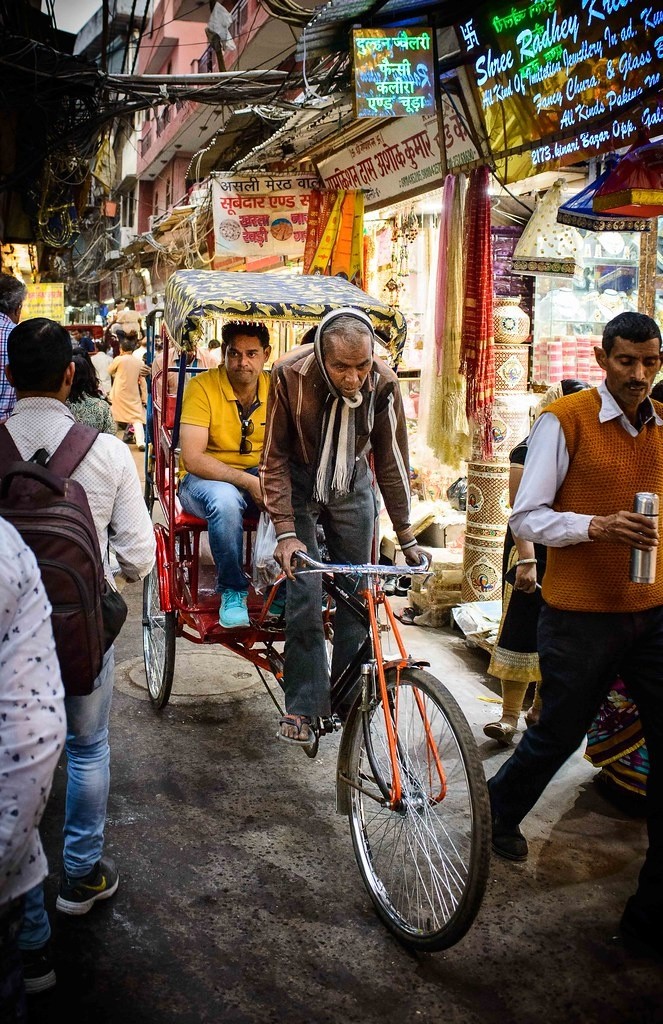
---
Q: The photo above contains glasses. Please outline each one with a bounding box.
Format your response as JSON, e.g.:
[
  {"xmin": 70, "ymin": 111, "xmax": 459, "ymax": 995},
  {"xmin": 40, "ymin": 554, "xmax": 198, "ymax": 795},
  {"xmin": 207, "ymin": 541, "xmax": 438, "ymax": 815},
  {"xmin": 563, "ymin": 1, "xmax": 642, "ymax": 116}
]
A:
[{"xmin": 239, "ymin": 419, "xmax": 254, "ymax": 454}]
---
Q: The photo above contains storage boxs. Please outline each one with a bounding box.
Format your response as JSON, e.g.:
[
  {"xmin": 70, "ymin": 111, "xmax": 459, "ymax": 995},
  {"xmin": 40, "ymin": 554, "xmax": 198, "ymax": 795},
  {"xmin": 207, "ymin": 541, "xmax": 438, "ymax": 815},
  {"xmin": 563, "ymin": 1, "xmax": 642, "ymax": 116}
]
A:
[{"xmin": 381, "ymin": 513, "xmax": 464, "ymax": 626}]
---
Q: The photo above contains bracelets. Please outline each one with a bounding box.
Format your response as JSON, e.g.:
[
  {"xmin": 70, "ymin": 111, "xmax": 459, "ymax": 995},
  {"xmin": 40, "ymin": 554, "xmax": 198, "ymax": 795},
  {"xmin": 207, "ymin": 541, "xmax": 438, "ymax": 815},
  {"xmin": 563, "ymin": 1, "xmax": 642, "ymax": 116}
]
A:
[{"xmin": 515, "ymin": 558, "xmax": 537, "ymax": 566}]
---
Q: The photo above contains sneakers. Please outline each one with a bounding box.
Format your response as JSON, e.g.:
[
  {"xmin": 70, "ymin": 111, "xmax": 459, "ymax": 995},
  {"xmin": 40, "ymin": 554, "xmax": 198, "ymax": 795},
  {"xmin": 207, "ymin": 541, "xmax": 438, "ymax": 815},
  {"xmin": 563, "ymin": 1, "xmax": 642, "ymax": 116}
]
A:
[
  {"xmin": 219, "ymin": 589, "xmax": 251, "ymax": 628},
  {"xmin": 23, "ymin": 945, "xmax": 56, "ymax": 993},
  {"xmin": 57, "ymin": 859, "xmax": 119, "ymax": 915},
  {"xmin": 263, "ymin": 584, "xmax": 337, "ymax": 616}
]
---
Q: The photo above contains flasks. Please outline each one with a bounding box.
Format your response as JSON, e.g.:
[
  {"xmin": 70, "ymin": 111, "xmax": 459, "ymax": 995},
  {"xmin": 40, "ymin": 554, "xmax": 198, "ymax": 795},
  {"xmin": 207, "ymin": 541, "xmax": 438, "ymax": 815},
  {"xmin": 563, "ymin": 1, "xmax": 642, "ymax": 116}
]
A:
[{"xmin": 631, "ymin": 491, "xmax": 658, "ymax": 584}]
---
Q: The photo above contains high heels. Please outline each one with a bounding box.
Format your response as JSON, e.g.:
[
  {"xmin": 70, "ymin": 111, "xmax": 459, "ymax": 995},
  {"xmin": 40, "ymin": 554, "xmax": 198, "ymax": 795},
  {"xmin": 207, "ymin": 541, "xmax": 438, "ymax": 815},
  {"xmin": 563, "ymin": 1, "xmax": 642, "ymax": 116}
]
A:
[
  {"xmin": 483, "ymin": 721, "xmax": 517, "ymax": 745},
  {"xmin": 524, "ymin": 715, "xmax": 539, "ymax": 728}
]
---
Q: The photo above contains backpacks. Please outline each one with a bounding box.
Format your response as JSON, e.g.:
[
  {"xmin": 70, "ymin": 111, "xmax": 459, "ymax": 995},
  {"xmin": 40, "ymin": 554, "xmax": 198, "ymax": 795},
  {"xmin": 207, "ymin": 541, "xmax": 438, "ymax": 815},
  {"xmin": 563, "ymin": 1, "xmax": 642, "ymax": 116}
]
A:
[{"xmin": 0, "ymin": 424, "xmax": 127, "ymax": 696}]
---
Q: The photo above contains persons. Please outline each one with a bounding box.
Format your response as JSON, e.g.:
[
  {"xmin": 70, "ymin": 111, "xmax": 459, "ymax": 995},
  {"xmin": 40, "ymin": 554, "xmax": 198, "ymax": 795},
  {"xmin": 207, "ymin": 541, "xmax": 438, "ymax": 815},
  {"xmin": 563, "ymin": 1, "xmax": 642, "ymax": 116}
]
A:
[
  {"xmin": 175, "ymin": 322, "xmax": 272, "ymax": 628},
  {"xmin": 0, "ymin": 270, "xmax": 221, "ymax": 1024},
  {"xmin": 482, "ymin": 312, "xmax": 663, "ymax": 964},
  {"xmin": 258, "ymin": 310, "xmax": 432, "ymax": 746}
]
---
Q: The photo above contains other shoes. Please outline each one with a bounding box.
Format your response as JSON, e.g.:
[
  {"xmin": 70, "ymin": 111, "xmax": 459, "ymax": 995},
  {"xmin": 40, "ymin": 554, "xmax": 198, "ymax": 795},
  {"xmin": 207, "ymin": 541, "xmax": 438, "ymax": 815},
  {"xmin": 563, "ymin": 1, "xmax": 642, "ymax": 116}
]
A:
[
  {"xmin": 396, "ymin": 575, "xmax": 413, "ymax": 595},
  {"xmin": 382, "ymin": 574, "xmax": 401, "ymax": 595},
  {"xmin": 618, "ymin": 896, "xmax": 662, "ymax": 960},
  {"xmin": 492, "ymin": 813, "xmax": 529, "ymax": 861},
  {"xmin": 123, "ymin": 426, "xmax": 131, "ymax": 440},
  {"xmin": 136, "ymin": 442, "xmax": 146, "ymax": 452}
]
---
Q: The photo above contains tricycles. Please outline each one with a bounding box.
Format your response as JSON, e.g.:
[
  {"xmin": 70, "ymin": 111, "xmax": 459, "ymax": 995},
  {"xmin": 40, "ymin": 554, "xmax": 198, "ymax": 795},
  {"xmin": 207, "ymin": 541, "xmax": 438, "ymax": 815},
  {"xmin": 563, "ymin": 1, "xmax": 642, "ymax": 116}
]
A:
[{"xmin": 135, "ymin": 268, "xmax": 492, "ymax": 950}]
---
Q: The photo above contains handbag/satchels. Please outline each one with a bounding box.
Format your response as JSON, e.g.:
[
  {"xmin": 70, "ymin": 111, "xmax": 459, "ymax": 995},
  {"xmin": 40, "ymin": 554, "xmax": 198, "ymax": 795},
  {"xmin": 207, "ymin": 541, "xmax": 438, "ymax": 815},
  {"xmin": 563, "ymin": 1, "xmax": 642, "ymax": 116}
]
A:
[{"xmin": 252, "ymin": 511, "xmax": 282, "ymax": 591}]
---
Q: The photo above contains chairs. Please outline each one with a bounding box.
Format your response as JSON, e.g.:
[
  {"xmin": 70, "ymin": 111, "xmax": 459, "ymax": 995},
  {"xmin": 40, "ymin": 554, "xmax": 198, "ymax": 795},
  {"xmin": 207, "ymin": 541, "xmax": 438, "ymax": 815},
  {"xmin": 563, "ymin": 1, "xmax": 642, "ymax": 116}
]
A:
[{"xmin": 152, "ymin": 367, "xmax": 260, "ymax": 605}]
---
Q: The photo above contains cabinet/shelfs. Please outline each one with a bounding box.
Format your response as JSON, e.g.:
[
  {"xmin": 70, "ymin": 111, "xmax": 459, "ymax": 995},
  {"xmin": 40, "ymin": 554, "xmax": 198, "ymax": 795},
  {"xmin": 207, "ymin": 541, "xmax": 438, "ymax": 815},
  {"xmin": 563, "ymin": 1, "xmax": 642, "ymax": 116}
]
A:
[{"xmin": 533, "ymin": 231, "xmax": 663, "ymax": 389}]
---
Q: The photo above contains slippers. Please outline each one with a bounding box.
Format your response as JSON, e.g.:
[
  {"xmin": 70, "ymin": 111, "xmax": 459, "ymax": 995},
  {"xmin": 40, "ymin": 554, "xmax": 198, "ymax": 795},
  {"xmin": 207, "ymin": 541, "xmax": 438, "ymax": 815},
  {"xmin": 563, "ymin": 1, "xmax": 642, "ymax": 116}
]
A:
[
  {"xmin": 277, "ymin": 715, "xmax": 313, "ymax": 746},
  {"xmin": 394, "ymin": 607, "xmax": 416, "ymax": 624}
]
